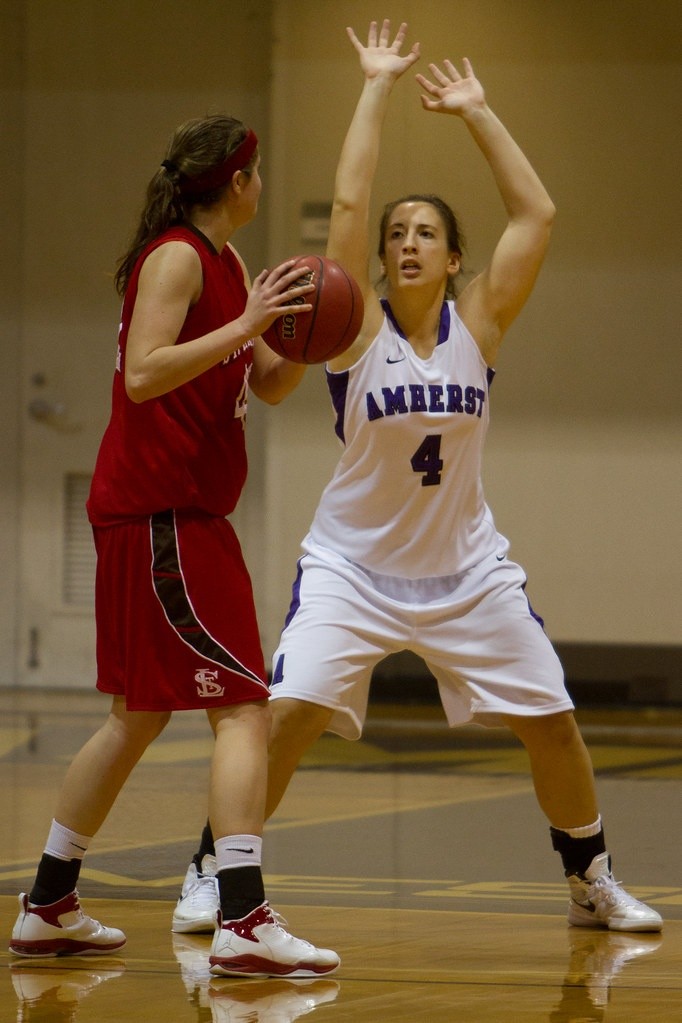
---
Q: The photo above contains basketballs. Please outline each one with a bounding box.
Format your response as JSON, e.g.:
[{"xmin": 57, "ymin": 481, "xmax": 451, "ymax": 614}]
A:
[{"xmin": 259, "ymin": 256, "xmax": 364, "ymax": 365}]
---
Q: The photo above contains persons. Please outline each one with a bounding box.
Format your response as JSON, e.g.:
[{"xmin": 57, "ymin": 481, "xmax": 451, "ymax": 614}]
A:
[
  {"xmin": 2, "ymin": 113, "xmax": 346, "ymax": 977},
  {"xmin": 172, "ymin": 16, "xmax": 667, "ymax": 941}
]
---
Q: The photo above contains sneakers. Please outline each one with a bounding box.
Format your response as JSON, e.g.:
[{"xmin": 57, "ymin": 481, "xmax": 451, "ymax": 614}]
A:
[
  {"xmin": 169, "ymin": 853, "xmax": 219, "ymax": 931},
  {"xmin": 169, "ymin": 933, "xmax": 213, "ymax": 974},
  {"xmin": 8, "ymin": 957, "xmax": 128, "ymax": 1022},
  {"xmin": 7, "ymin": 886, "xmax": 128, "ymax": 956},
  {"xmin": 567, "ymin": 927, "xmax": 664, "ymax": 977},
  {"xmin": 566, "ymin": 852, "xmax": 664, "ymax": 930},
  {"xmin": 209, "ymin": 899, "xmax": 341, "ymax": 978},
  {"xmin": 208, "ymin": 978, "xmax": 340, "ymax": 1023}
]
